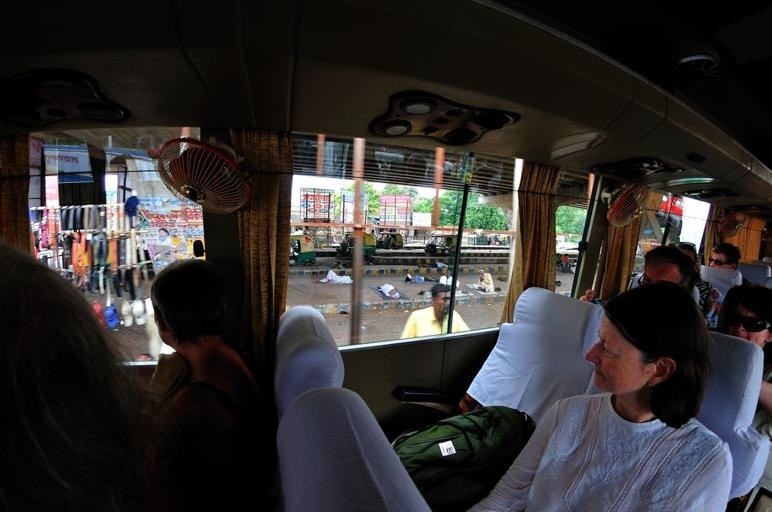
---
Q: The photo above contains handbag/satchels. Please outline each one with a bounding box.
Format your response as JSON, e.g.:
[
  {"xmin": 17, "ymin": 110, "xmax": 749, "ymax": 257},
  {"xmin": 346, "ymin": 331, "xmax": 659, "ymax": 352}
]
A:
[{"xmin": 391, "ymin": 406, "xmax": 536, "ymax": 512}]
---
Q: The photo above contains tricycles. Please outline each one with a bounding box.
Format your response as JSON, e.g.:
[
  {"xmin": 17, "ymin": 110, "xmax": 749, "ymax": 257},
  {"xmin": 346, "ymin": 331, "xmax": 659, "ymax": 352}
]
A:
[
  {"xmin": 333, "ymin": 231, "xmax": 378, "ymax": 259},
  {"xmin": 377, "ymin": 231, "xmax": 404, "ymax": 250},
  {"xmin": 467, "ymin": 232, "xmax": 487, "ymax": 245},
  {"xmin": 422, "ymin": 232, "xmax": 457, "ymax": 255},
  {"xmin": 288, "ymin": 232, "xmax": 316, "ymax": 267}
]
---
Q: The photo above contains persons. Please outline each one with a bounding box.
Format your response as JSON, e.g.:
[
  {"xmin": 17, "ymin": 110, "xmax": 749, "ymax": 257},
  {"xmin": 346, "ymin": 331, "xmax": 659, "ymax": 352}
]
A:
[
  {"xmin": 145, "ymin": 257, "xmax": 264, "ymax": 512},
  {"xmin": 641, "ymin": 244, "xmax": 700, "ymax": 296},
  {"xmin": 157, "ymin": 226, "xmax": 170, "ymax": 242},
  {"xmin": 715, "ymin": 281, "xmax": 772, "ymax": 419},
  {"xmin": 561, "ymin": 254, "xmax": 576, "ymax": 267},
  {"xmin": 707, "ymin": 242, "xmax": 743, "ymax": 274},
  {"xmin": 399, "ymin": 284, "xmax": 472, "ymax": 339},
  {"xmin": 0, "ymin": 249, "xmax": 171, "ymax": 511},
  {"xmin": 464, "ymin": 279, "xmax": 734, "ymax": 512},
  {"xmin": 291, "ymin": 214, "xmax": 501, "ymax": 304}
]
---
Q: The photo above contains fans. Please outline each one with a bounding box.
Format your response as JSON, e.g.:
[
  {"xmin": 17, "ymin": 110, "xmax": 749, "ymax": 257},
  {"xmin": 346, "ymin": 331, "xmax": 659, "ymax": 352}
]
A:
[
  {"xmin": 599, "ymin": 175, "xmax": 650, "ymax": 229},
  {"xmin": 157, "ymin": 139, "xmax": 253, "ymax": 213},
  {"xmin": 713, "ymin": 204, "xmax": 747, "ymax": 238}
]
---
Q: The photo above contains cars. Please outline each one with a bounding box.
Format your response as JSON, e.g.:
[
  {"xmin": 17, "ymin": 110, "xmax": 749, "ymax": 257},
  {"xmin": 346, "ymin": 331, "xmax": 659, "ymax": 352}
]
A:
[{"xmin": 556, "ymin": 244, "xmax": 580, "ymax": 254}]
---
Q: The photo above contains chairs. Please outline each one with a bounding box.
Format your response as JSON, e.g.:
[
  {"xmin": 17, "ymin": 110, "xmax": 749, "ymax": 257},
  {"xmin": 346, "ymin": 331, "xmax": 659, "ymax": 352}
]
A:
[
  {"xmin": 274, "ymin": 306, "xmax": 347, "ymax": 415},
  {"xmin": 374, "ymin": 286, "xmax": 609, "ymax": 504},
  {"xmin": 277, "ymin": 392, "xmax": 432, "ymax": 512},
  {"xmin": 587, "ymin": 256, "xmax": 771, "ymax": 511}
]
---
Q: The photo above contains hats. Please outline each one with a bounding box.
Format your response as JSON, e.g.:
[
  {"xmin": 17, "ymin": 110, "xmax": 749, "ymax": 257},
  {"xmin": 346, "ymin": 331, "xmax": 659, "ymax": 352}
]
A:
[{"xmin": 93, "ymin": 298, "xmax": 154, "ymax": 328}]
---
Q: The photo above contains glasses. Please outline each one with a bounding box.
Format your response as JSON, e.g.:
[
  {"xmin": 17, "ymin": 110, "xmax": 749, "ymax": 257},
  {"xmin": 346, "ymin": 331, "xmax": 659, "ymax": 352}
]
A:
[
  {"xmin": 708, "ymin": 255, "xmax": 729, "ymax": 265},
  {"xmin": 722, "ymin": 309, "xmax": 772, "ymax": 332}
]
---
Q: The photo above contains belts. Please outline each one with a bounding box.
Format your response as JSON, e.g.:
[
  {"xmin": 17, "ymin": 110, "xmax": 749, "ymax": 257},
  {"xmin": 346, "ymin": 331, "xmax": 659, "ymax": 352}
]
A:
[{"xmin": 34, "ymin": 204, "xmax": 138, "ymax": 306}]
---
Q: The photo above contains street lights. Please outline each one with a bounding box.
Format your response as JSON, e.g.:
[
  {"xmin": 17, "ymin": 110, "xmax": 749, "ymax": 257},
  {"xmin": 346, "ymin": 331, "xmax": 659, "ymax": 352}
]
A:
[{"xmin": 108, "ymin": 154, "xmax": 129, "ymax": 202}]
